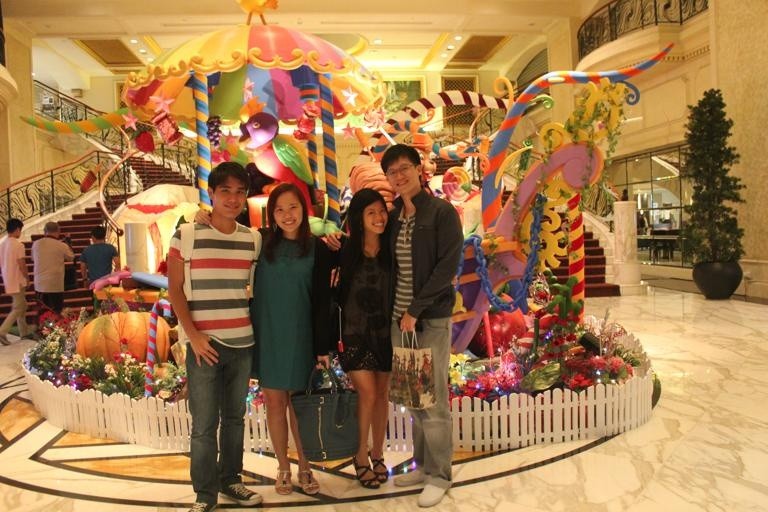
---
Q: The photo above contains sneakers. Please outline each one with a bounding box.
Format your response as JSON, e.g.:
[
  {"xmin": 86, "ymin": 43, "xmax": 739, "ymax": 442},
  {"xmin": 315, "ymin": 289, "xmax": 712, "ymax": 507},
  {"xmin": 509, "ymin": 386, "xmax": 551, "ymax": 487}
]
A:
[
  {"xmin": 393, "ymin": 469, "xmax": 427, "ymax": 487},
  {"xmin": 218, "ymin": 483, "xmax": 263, "ymax": 506},
  {"xmin": 189, "ymin": 502, "xmax": 217, "ymax": 512},
  {"xmin": 417, "ymin": 483, "xmax": 452, "ymax": 507}
]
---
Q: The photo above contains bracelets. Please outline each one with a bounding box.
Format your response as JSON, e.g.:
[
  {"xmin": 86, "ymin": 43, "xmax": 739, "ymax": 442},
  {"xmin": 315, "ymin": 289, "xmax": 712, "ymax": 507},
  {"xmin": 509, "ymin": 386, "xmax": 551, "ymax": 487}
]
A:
[{"xmin": 83, "ymin": 277, "xmax": 88, "ymax": 281}]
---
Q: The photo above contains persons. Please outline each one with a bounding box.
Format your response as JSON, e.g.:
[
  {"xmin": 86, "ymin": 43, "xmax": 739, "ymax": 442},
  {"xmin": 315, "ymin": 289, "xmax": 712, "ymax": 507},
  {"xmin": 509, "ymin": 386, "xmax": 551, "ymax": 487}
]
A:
[
  {"xmin": 327, "ymin": 143, "xmax": 467, "ymax": 507},
  {"xmin": 0, "ymin": 218, "xmax": 36, "ymax": 345},
  {"xmin": 323, "ymin": 187, "xmax": 460, "ymax": 490},
  {"xmin": 193, "ymin": 182, "xmax": 334, "ymax": 497},
  {"xmin": 31, "ymin": 222, "xmax": 75, "ymax": 327},
  {"xmin": 166, "ymin": 160, "xmax": 266, "ymax": 510},
  {"xmin": 78, "ymin": 226, "xmax": 121, "ymax": 310}
]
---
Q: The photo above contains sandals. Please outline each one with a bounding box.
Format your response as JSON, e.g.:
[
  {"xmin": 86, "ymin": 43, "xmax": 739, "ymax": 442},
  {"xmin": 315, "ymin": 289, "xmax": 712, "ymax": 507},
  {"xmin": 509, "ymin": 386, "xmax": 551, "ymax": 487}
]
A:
[
  {"xmin": 275, "ymin": 470, "xmax": 293, "ymax": 495},
  {"xmin": 353, "ymin": 456, "xmax": 380, "ymax": 489},
  {"xmin": 371, "ymin": 458, "xmax": 389, "ymax": 483},
  {"xmin": 299, "ymin": 470, "xmax": 319, "ymax": 495}
]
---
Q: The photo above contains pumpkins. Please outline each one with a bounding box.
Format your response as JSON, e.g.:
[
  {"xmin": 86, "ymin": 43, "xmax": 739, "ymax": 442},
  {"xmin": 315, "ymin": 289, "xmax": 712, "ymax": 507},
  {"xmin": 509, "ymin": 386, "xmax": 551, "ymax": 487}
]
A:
[{"xmin": 76, "ymin": 311, "xmax": 172, "ymax": 363}]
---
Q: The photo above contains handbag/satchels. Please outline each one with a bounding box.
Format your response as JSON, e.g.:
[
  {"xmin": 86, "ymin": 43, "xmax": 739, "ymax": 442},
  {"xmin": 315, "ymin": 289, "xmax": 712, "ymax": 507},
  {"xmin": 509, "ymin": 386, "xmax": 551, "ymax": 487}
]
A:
[
  {"xmin": 388, "ymin": 328, "xmax": 437, "ymax": 410},
  {"xmin": 291, "ymin": 361, "xmax": 361, "ymax": 462}
]
